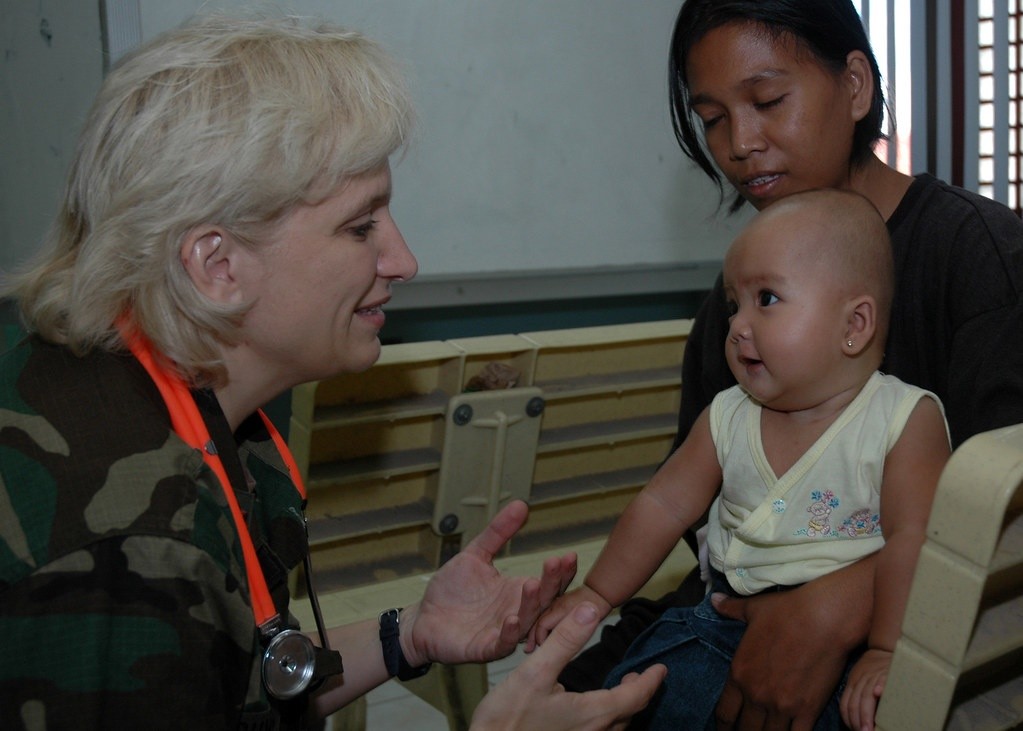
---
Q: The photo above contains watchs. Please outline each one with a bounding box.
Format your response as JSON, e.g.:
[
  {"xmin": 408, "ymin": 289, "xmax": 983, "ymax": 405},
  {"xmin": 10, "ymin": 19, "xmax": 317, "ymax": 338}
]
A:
[{"xmin": 378, "ymin": 606, "xmax": 431, "ymax": 684}]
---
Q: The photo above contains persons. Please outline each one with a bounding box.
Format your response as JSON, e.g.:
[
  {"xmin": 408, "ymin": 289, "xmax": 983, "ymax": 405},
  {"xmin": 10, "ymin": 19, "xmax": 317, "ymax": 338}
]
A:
[
  {"xmin": 518, "ymin": 187, "xmax": 953, "ymax": 731},
  {"xmin": 3, "ymin": 18, "xmax": 579, "ymax": 730},
  {"xmin": 551, "ymin": 0, "xmax": 1022, "ymax": 731}
]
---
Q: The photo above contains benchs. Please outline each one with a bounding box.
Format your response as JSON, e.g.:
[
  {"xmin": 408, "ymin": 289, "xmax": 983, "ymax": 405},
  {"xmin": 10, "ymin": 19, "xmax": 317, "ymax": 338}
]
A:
[{"xmin": 880, "ymin": 420, "xmax": 1023, "ymax": 731}]
